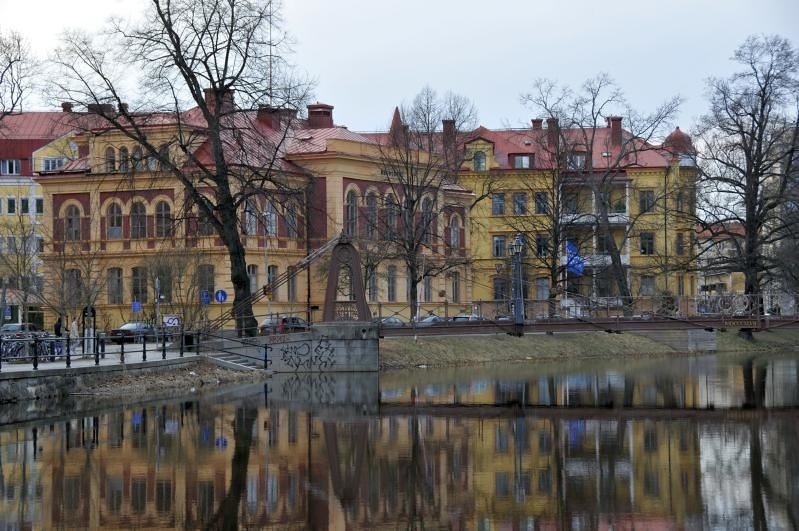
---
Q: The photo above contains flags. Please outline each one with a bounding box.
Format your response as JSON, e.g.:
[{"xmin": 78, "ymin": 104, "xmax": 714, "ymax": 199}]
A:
[{"xmin": 566, "ymin": 241, "xmax": 584, "ymax": 276}]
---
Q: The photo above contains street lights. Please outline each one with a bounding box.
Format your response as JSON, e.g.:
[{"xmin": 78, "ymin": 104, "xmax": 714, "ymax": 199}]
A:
[{"xmin": 507, "ymin": 236, "xmax": 524, "ymax": 320}]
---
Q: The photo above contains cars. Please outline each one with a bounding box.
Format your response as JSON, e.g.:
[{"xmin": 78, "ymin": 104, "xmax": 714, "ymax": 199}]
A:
[
  {"xmin": 373, "ymin": 317, "xmax": 404, "ymax": 323},
  {"xmin": 257, "ymin": 316, "xmax": 308, "ymax": 336},
  {"xmin": 110, "ymin": 323, "xmax": 152, "ymax": 345},
  {"xmin": 1, "ymin": 323, "xmax": 48, "ymax": 337}
]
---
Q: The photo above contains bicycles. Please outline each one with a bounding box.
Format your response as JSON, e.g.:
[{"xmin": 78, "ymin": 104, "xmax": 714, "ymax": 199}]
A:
[{"xmin": 0, "ymin": 332, "xmax": 63, "ymax": 362}]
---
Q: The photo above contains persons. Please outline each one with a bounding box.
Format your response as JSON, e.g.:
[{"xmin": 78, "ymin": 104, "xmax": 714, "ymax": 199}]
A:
[{"xmin": 54, "ymin": 315, "xmax": 80, "ymax": 356}]
